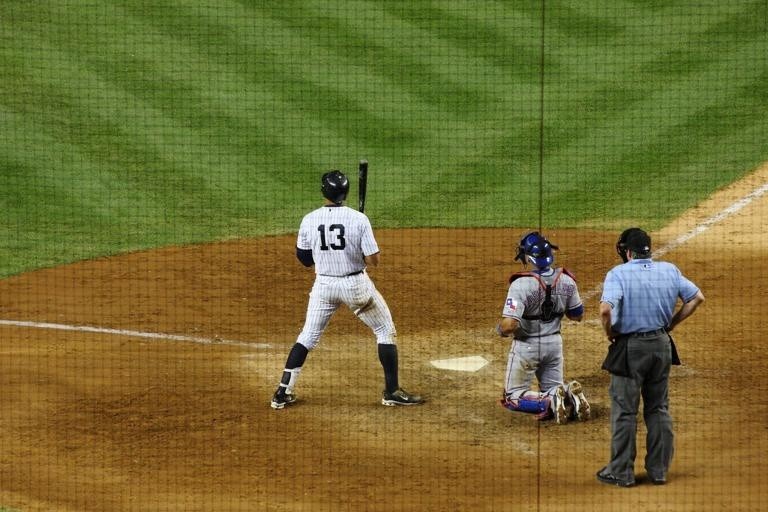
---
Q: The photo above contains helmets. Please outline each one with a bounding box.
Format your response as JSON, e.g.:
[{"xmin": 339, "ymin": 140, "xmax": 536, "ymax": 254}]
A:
[
  {"xmin": 515, "ymin": 232, "xmax": 560, "ymax": 268},
  {"xmin": 321, "ymin": 170, "xmax": 349, "ymax": 205},
  {"xmin": 616, "ymin": 227, "xmax": 650, "ymax": 262}
]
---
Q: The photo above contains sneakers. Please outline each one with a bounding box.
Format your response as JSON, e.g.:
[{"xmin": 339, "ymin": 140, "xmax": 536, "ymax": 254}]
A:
[
  {"xmin": 597, "ymin": 467, "xmax": 635, "ymax": 487},
  {"xmin": 567, "ymin": 380, "xmax": 591, "ymax": 422},
  {"xmin": 652, "ymin": 475, "xmax": 666, "ymax": 484},
  {"xmin": 549, "ymin": 386, "xmax": 569, "ymax": 425},
  {"xmin": 271, "ymin": 392, "xmax": 298, "ymax": 410},
  {"xmin": 381, "ymin": 388, "xmax": 423, "ymax": 407}
]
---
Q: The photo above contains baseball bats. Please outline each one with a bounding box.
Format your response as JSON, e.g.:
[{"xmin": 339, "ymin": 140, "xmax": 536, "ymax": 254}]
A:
[{"xmin": 357, "ymin": 160, "xmax": 369, "ymax": 213}]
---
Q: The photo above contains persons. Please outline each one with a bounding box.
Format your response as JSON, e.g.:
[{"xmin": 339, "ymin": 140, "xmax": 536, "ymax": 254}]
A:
[
  {"xmin": 496, "ymin": 231, "xmax": 591, "ymax": 426},
  {"xmin": 270, "ymin": 170, "xmax": 424, "ymax": 410},
  {"xmin": 595, "ymin": 227, "xmax": 706, "ymax": 488}
]
